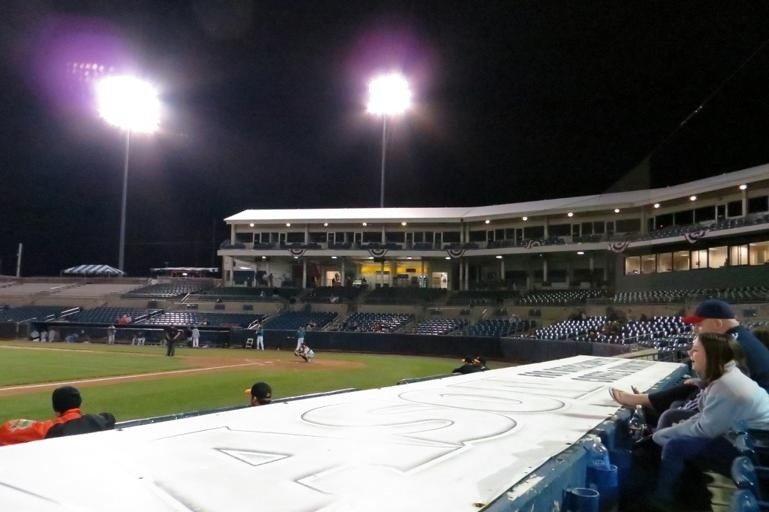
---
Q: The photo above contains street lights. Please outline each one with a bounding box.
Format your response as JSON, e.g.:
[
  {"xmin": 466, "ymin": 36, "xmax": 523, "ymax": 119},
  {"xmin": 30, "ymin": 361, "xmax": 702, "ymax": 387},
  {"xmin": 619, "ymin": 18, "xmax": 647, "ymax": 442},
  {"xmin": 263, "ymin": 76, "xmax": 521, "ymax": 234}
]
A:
[
  {"xmin": 366, "ymin": 70, "xmax": 412, "ymax": 208},
  {"xmin": 97, "ymin": 71, "xmax": 163, "ymax": 275}
]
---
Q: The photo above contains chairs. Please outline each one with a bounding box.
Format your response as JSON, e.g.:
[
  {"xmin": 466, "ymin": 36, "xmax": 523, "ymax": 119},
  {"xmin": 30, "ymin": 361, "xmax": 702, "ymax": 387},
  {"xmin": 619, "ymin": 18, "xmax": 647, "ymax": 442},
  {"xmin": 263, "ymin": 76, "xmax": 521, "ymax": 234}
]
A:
[
  {"xmin": 124, "ymin": 281, "xmax": 211, "ymax": 298},
  {"xmin": 516, "ymin": 285, "xmax": 769, "ymax": 307},
  {"xmin": 701, "ymin": 429, "xmax": 769, "ymax": 512}
]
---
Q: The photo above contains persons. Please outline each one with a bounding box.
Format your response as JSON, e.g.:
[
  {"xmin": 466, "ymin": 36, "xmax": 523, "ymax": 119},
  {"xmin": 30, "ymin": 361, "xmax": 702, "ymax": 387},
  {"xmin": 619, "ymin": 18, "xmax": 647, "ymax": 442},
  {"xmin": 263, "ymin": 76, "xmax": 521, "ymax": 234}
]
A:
[
  {"xmin": 571, "ymin": 279, "xmax": 616, "ymax": 302},
  {"xmin": 0, "ymin": 386, "xmax": 115, "ymax": 445},
  {"xmin": 294, "ymin": 326, "xmax": 314, "ymax": 362},
  {"xmin": 566, "ymin": 310, "xmax": 590, "ymax": 322},
  {"xmin": 307, "ymin": 320, "xmax": 315, "ymax": 330},
  {"xmin": 31, "ymin": 328, "xmax": 90, "ymax": 344},
  {"xmin": 454, "ymin": 356, "xmax": 489, "ymax": 374},
  {"xmin": 257, "ymin": 272, "xmax": 281, "ymax": 297},
  {"xmin": 311, "ymin": 273, "xmax": 368, "ymax": 297},
  {"xmin": 478, "ymin": 303, "xmax": 542, "ymax": 330},
  {"xmin": 164, "ymin": 323, "xmax": 181, "ymax": 357},
  {"xmin": 192, "ymin": 325, "xmax": 199, "ymax": 348},
  {"xmin": 224, "ymin": 326, "xmax": 265, "ymax": 349},
  {"xmin": 375, "ymin": 323, "xmax": 383, "ymax": 333},
  {"xmin": 474, "ymin": 278, "xmax": 552, "ymax": 292},
  {"xmin": 586, "ymin": 305, "xmax": 648, "ymax": 342},
  {"xmin": 107, "ymin": 315, "xmax": 146, "ymax": 345},
  {"xmin": 607, "ymin": 299, "xmax": 768, "ymax": 511},
  {"xmin": 244, "ymin": 382, "xmax": 272, "ymax": 406},
  {"xmin": 431, "ymin": 304, "xmax": 472, "ymax": 317},
  {"xmin": 203, "ymin": 318, "xmax": 208, "ymax": 325}
]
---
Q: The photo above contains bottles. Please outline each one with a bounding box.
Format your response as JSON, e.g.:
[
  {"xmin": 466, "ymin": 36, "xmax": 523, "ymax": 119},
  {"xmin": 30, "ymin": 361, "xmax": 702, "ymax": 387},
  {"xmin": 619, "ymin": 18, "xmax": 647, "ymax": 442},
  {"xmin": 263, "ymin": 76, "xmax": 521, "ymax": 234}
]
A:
[
  {"xmin": 625, "ymin": 414, "xmax": 640, "ymax": 445},
  {"xmin": 592, "ymin": 436, "xmax": 612, "ymax": 473},
  {"xmin": 633, "ymin": 405, "xmax": 648, "ymax": 435}
]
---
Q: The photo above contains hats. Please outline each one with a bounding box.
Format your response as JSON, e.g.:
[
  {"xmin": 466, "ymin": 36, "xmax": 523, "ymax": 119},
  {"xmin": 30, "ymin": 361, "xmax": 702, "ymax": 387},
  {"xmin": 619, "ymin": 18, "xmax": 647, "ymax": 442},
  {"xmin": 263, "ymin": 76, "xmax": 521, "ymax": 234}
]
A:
[
  {"xmin": 245, "ymin": 383, "xmax": 272, "ymax": 398},
  {"xmin": 53, "ymin": 386, "xmax": 81, "ymax": 411},
  {"xmin": 682, "ymin": 300, "xmax": 734, "ymax": 322},
  {"xmin": 462, "ymin": 355, "xmax": 481, "ymax": 363}
]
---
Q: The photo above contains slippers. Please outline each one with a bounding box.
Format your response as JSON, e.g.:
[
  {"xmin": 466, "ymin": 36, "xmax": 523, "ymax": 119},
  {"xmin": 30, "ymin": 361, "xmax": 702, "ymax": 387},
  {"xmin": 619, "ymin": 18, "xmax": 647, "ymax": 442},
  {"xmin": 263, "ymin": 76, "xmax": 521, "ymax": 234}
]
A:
[{"xmin": 609, "ymin": 385, "xmax": 640, "ymax": 409}]
